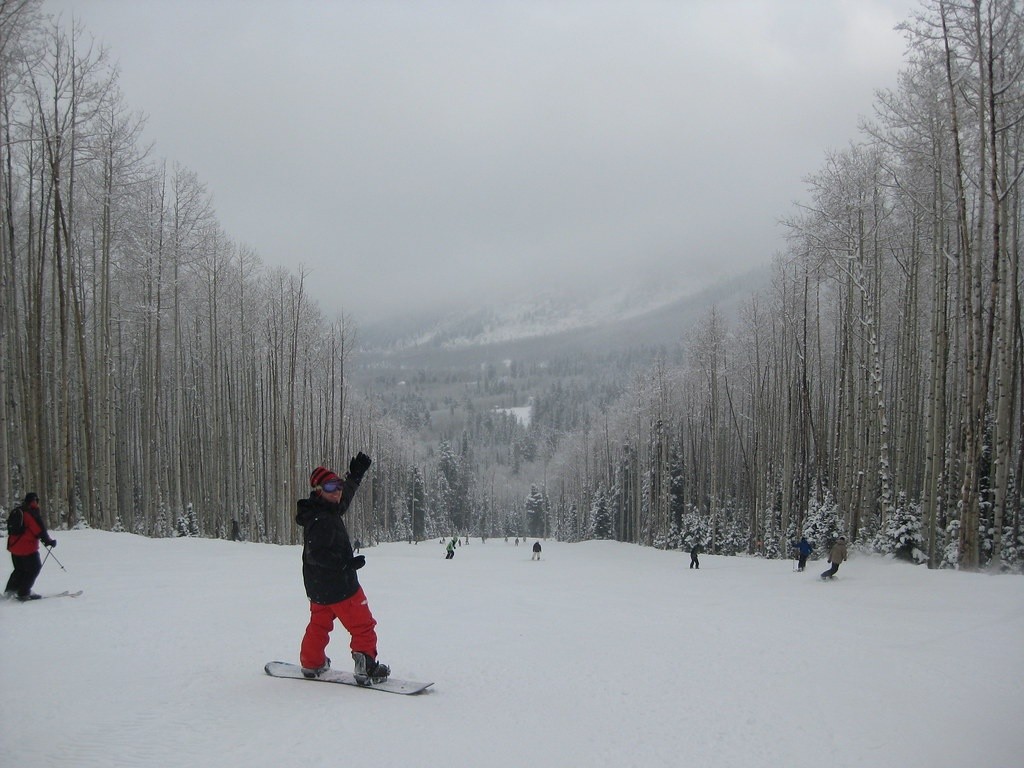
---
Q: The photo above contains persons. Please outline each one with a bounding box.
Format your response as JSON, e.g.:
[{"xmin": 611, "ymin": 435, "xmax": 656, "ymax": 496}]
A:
[
  {"xmin": 690, "ymin": 544, "xmax": 699, "ymax": 570},
  {"xmin": 531, "ymin": 541, "xmax": 541, "ymax": 560},
  {"xmin": 515, "ymin": 538, "xmax": 520, "ymax": 546},
  {"xmin": 523, "ymin": 536, "xmax": 526, "ymax": 542},
  {"xmin": 505, "ymin": 535, "xmax": 508, "ymax": 542},
  {"xmin": 230, "ymin": 518, "xmax": 242, "ymax": 542},
  {"xmin": 295, "ymin": 452, "xmax": 391, "ymax": 686},
  {"xmin": 353, "ymin": 539, "xmax": 360, "ymax": 553},
  {"xmin": 3, "ymin": 491, "xmax": 57, "ymax": 601},
  {"xmin": 820, "ymin": 537, "xmax": 848, "ymax": 581},
  {"xmin": 465, "ymin": 533, "xmax": 469, "ymax": 545},
  {"xmin": 482, "ymin": 536, "xmax": 486, "ymax": 543},
  {"xmin": 792, "ymin": 537, "xmax": 813, "ymax": 572},
  {"xmin": 445, "ymin": 536, "xmax": 458, "ymax": 559}
]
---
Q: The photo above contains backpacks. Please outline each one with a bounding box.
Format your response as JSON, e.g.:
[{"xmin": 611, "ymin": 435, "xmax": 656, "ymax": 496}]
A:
[{"xmin": 7, "ymin": 507, "xmax": 24, "ymax": 535}]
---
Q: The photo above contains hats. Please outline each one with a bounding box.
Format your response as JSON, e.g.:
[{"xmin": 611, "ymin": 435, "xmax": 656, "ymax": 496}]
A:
[{"xmin": 310, "ymin": 467, "xmax": 339, "ymax": 498}]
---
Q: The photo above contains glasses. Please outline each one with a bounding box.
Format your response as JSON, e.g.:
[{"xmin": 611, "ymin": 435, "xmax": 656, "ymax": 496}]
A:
[
  {"xmin": 322, "ymin": 479, "xmax": 345, "ymax": 493},
  {"xmin": 34, "ymin": 498, "xmax": 39, "ymax": 503}
]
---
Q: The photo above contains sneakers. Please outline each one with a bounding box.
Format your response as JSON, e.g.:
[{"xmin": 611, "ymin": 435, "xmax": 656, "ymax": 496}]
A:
[
  {"xmin": 357, "ymin": 656, "xmax": 391, "ymax": 686},
  {"xmin": 303, "ymin": 658, "xmax": 331, "ymax": 678}
]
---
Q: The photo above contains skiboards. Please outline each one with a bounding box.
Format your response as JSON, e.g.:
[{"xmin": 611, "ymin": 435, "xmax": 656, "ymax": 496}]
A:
[{"xmin": 41, "ymin": 589, "xmax": 84, "ymax": 600}]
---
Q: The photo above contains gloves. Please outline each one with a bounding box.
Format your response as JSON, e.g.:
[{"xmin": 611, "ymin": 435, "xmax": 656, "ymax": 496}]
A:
[
  {"xmin": 347, "ymin": 452, "xmax": 371, "ymax": 484},
  {"xmin": 49, "ymin": 540, "xmax": 56, "ymax": 547},
  {"xmin": 345, "ymin": 555, "xmax": 366, "ymax": 571}
]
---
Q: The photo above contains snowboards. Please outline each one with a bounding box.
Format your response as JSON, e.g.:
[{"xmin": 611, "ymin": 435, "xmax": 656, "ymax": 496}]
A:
[{"xmin": 264, "ymin": 661, "xmax": 435, "ymax": 696}]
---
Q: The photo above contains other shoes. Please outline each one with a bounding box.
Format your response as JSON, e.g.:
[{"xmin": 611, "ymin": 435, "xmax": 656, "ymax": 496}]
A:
[{"xmin": 19, "ymin": 593, "xmax": 41, "ymax": 600}]
